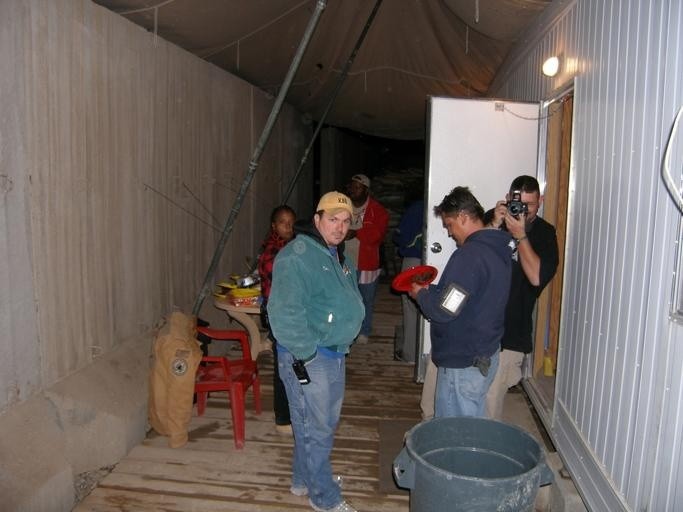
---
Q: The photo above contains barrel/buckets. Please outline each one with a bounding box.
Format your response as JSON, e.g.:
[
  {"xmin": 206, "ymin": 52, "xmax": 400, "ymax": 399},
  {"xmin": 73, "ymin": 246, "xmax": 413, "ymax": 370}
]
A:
[{"xmin": 394, "ymin": 415, "xmax": 555, "ymax": 512}]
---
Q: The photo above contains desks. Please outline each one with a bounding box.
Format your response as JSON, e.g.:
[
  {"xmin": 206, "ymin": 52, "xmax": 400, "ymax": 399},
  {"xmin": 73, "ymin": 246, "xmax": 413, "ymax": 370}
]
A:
[{"xmin": 213, "ymin": 297, "xmax": 275, "ymax": 362}]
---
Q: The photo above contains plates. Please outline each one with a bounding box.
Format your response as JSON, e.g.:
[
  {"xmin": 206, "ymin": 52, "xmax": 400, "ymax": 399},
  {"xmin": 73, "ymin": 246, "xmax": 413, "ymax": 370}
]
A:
[{"xmin": 391, "ymin": 265, "xmax": 438, "ymax": 292}]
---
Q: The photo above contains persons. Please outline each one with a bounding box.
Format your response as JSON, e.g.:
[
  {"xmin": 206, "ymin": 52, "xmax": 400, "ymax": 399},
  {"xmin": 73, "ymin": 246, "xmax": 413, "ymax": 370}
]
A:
[
  {"xmin": 259, "ymin": 205, "xmax": 297, "ymax": 425},
  {"xmin": 338, "ymin": 173, "xmax": 389, "ymax": 345},
  {"xmin": 397, "ymin": 200, "xmax": 425, "ymax": 368},
  {"xmin": 406, "ymin": 186, "xmax": 512, "ymax": 422},
  {"xmin": 416, "ymin": 175, "xmax": 558, "ymax": 422},
  {"xmin": 264, "ymin": 191, "xmax": 368, "ymax": 511}
]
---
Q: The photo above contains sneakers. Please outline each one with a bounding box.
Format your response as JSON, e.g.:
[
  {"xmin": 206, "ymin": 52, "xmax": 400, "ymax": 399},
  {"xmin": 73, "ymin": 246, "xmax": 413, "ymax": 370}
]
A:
[
  {"xmin": 309, "ymin": 497, "xmax": 356, "ymax": 512},
  {"xmin": 290, "ymin": 473, "xmax": 343, "ymax": 496},
  {"xmin": 275, "ymin": 423, "xmax": 292, "ymax": 435},
  {"xmin": 357, "ymin": 334, "xmax": 368, "ymax": 345}
]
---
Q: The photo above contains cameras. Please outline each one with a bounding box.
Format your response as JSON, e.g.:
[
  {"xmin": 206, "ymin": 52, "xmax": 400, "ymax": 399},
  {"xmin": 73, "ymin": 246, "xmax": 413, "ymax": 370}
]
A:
[{"xmin": 501, "ymin": 190, "xmax": 529, "ymax": 219}]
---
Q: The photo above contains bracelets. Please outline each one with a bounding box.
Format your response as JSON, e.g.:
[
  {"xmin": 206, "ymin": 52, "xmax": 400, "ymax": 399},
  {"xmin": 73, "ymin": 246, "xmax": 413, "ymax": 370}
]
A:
[{"xmin": 514, "ymin": 235, "xmax": 529, "ymax": 245}]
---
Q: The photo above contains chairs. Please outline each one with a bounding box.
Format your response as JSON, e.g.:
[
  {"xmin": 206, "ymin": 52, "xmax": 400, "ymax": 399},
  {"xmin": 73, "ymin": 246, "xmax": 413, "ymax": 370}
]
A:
[{"xmin": 193, "ymin": 325, "xmax": 261, "ymax": 448}]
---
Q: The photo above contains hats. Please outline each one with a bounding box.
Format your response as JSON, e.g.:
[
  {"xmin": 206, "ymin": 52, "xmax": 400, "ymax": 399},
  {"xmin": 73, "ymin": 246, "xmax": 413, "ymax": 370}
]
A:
[
  {"xmin": 316, "ymin": 190, "xmax": 353, "ymax": 217},
  {"xmin": 351, "ymin": 173, "xmax": 371, "ymax": 188}
]
